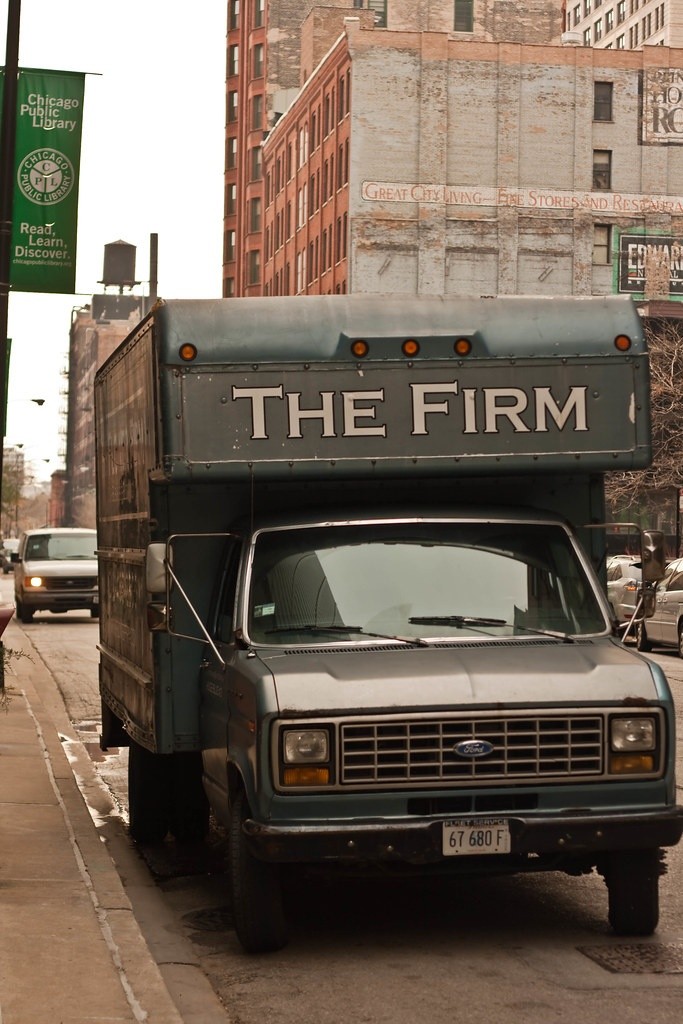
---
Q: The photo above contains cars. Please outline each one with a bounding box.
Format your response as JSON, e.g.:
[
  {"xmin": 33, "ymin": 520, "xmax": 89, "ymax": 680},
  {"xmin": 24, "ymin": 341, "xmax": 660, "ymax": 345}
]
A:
[
  {"xmin": 0, "ymin": 538, "xmax": 20, "ymax": 573},
  {"xmin": 608, "ymin": 555, "xmax": 683, "ymax": 659}
]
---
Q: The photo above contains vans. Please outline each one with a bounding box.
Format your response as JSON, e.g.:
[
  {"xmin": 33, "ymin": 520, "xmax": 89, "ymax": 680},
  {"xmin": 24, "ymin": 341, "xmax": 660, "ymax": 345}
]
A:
[{"xmin": 15, "ymin": 528, "xmax": 100, "ymax": 623}]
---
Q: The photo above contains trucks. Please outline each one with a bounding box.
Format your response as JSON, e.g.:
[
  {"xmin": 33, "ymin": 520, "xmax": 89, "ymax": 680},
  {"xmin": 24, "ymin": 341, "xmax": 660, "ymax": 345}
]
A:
[{"xmin": 93, "ymin": 294, "xmax": 683, "ymax": 955}]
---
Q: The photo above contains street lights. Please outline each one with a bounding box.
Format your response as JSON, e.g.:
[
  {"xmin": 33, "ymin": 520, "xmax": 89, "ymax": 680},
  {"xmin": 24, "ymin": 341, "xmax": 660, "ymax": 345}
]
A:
[{"xmin": 15, "ymin": 475, "xmax": 34, "ymax": 528}]
